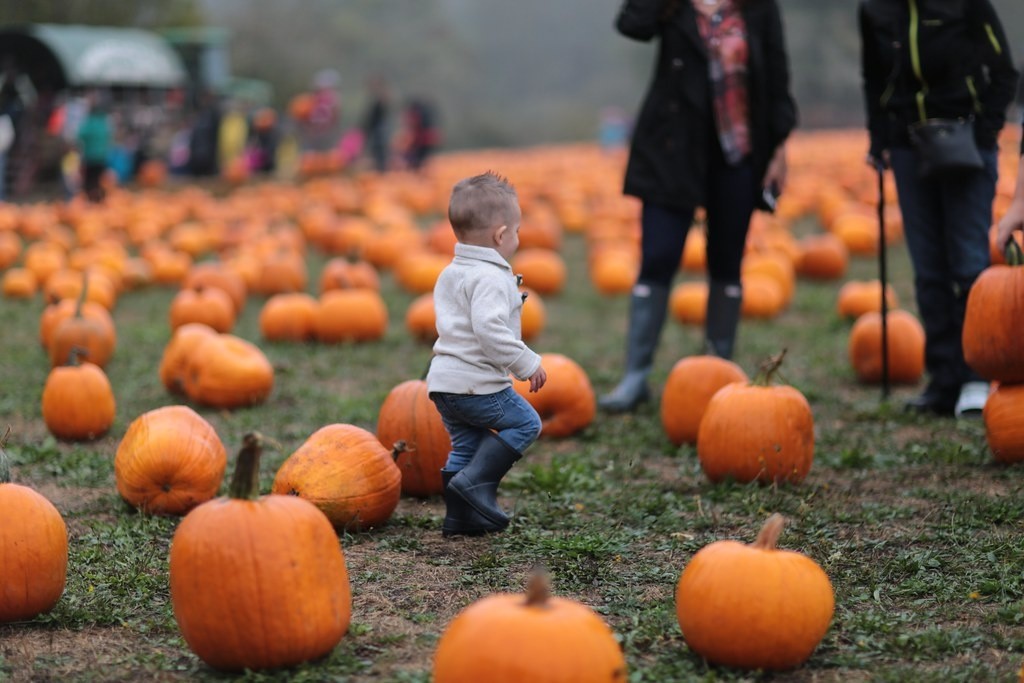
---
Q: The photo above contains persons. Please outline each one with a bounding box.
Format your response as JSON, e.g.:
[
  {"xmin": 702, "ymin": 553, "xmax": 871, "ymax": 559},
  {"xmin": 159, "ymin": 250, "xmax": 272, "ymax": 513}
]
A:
[
  {"xmin": 424, "ymin": 171, "xmax": 549, "ymax": 534},
  {"xmin": 6, "ymin": 67, "xmax": 443, "ymax": 201},
  {"xmin": 993, "ymin": 122, "xmax": 1024, "ymax": 262},
  {"xmin": 853, "ymin": 1, "xmax": 1016, "ymax": 412},
  {"xmin": 592, "ymin": 0, "xmax": 801, "ymax": 417}
]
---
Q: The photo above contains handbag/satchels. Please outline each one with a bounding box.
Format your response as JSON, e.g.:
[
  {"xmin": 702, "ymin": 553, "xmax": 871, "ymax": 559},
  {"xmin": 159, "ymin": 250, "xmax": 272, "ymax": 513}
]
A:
[{"xmin": 905, "ymin": 113, "xmax": 986, "ymax": 187}]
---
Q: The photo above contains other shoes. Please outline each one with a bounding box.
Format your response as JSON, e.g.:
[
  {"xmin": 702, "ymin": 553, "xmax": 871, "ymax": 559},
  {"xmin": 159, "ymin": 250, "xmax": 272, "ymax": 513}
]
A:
[{"xmin": 896, "ymin": 376, "xmax": 954, "ymax": 415}]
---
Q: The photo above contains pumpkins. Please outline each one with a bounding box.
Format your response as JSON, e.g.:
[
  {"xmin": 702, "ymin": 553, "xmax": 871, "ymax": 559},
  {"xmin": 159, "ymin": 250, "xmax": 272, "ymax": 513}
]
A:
[{"xmin": 5, "ymin": 124, "xmax": 1024, "ymax": 683}]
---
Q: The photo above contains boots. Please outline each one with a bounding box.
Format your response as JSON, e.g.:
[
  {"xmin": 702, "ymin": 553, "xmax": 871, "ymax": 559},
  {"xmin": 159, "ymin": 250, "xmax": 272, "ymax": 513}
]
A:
[
  {"xmin": 597, "ymin": 282, "xmax": 670, "ymax": 413},
  {"xmin": 440, "ymin": 467, "xmax": 505, "ymax": 538},
  {"xmin": 447, "ymin": 428, "xmax": 524, "ymax": 529},
  {"xmin": 699, "ymin": 282, "xmax": 743, "ymax": 364}
]
